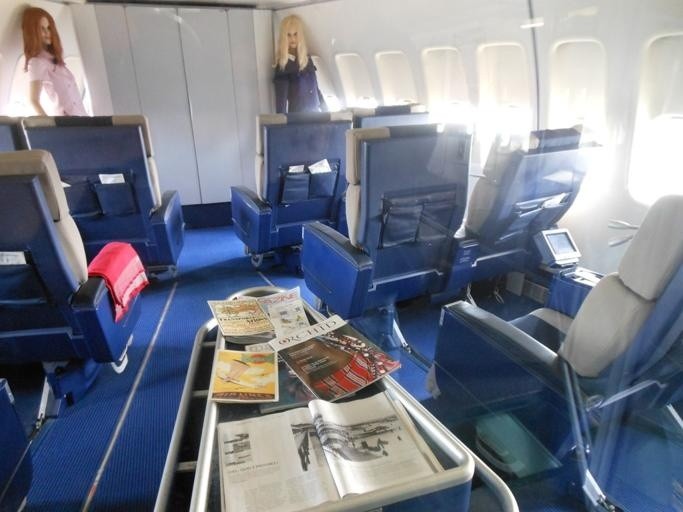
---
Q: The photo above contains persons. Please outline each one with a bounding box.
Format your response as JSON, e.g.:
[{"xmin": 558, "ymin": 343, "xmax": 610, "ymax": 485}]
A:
[
  {"xmin": 271, "ymin": 14, "xmax": 330, "ymax": 115},
  {"xmin": 21, "ymin": 6, "xmax": 89, "ymax": 116}
]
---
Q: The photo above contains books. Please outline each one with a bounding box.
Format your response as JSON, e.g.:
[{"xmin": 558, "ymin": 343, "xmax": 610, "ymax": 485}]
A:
[
  {"xmin": 208, "ymin": 314, "xmax": 401, "ymax": 414},
  {"xmin": 207, "ymin": 298, "xmax": 275, "ymax": 344},
  {"xmin": 217, "ymin": 390, "xmax": 446, "ymax": 512}
]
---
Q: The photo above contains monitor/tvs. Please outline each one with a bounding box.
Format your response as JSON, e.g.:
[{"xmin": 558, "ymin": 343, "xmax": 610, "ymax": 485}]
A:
[{"xmin": 545, "ymin": 232, "xmax": 576, "ymax": 256}]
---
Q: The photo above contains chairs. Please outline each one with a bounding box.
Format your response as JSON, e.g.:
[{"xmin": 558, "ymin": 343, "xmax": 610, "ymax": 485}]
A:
[
  {"xmin": 0, "ymin": 150, "xmax": 152, "ymax": 420},
  {"xmin": 230, "ymin": 103, "xmax": 428, "ymax": 280},
  {"xmin": 1, "ymin": 382, "xmax": 38, "ymax": 510},
  {"xmin": 300, "ymin": 124, "xmax": 587, "ymax": 354},
  {"xmin": 0, "ymin": 112, "xmax": 187, "ymax": 273},
  {"xmin": 427, "ymin": 191, "xmax": 683, "ymax": 511}
]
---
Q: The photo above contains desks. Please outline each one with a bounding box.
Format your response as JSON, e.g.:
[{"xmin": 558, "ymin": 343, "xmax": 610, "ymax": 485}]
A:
[{"xmin": 150, "ymin": 287, "xmax": 521, "ymax": 512}]
